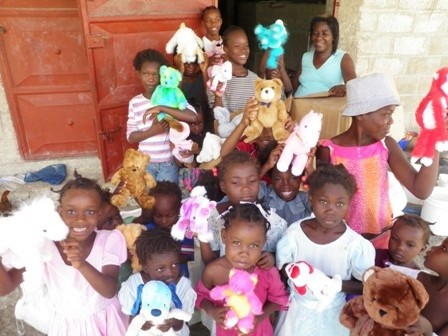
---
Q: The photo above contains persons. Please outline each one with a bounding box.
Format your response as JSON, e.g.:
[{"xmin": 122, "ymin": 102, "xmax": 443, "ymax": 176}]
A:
[
  {"xmin": 216, "ymin": 144, "xmax": 315, "ymax": 227},
  {"xmin": 218, "ymin": 97, "xmax": 294, "ymax": 159},
  {"xmin": 205, "ymin": 22, "xmax": 261, "ymax": 112},
  {"xmin": 293, "ymin": 13, "xmax": 357, "ymax": 99},
  {"xmin": 172, "ymin": 44, "xmax": 210, "ymax": 98},
  {"xmin": 414, "ymin": 236, "xmax": 448, "ymax": 336},
  {"xmin": 118, "ymin": 227, "xmax": 198, "ymax": 336},
  {"xmin": 375, "ymin": 213, "xmax": 437, "ymax": 271},
  {"xmin": 271, "ymin": 163, "xmax": 377, "ymax": 336},
  {"xmin": 201, "ymin": 5, "xmax": 228, "ymax": 88},
  {"xmin": 169, "ymin": 103, "xmax": 213, "ymax": 168},
  {"xmin": 315, "ymin": 70, "xmax": 448, "ymax": 248},
  {"xmin": 200, "ymin": 203, "xmax": 290, "ymax": 336},
  {"xmin": 149, "ymin": 179, "xmax": 182, "ymax": 231},
  {"xmin": 96, "ymin": 180, "xmax": 154, "ymax": 293},
  {"xmin": 125, "ymin": 47, "xmax": 199, "ymax": 184},
  {"xmin": 259, "ymin": 49, "xmax": 294, "ymax": 101},
  {"xmin": 0, "ymin": 167, "xmax": 132, "ymax": 336},
  {"xmin": 200, "ymin": 151, "xmax": 261, "ymax": 266}
]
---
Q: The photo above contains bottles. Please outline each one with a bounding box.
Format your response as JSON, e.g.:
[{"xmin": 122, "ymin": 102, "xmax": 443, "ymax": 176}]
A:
[{"xmin": 340, "ymin": 72, "xmax": 401, "ymax": 115}]
